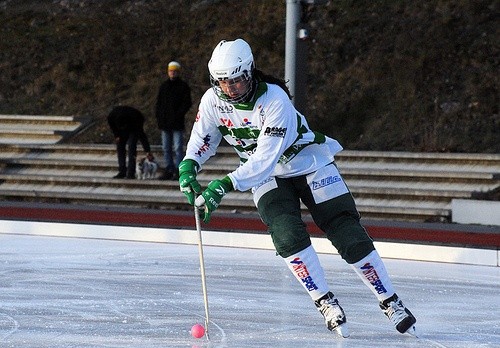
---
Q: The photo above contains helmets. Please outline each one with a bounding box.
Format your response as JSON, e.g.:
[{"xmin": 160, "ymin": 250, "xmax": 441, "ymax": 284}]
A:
[{"xmin": 208, "ymin": 37, "xmax": 256, "ymax": 81}]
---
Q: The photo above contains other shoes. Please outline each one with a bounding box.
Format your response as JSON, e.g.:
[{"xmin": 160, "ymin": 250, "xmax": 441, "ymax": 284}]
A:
[
  {"xmin": 158, "ymin": 171, "xmax": 173, "ymax": 181},
  {"xmin": 171, "ymin": 170, "xmax": 179, "ymax": 180},
  {"xmin": 125, "ymin": 173, "xmax": 136, "ymax": 180},
  {"xmin": 113, "ymin": 170, "xmax": 126, "ymax": 179}
]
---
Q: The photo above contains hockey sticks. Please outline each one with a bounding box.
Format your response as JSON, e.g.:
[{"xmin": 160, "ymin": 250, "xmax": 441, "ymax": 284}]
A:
[{"xmin": 194, "ymin": 194, "xmax": 212, "ymax": 342}]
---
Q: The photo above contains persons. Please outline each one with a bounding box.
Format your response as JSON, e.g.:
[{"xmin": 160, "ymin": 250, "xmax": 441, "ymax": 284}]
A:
[
  {"xmin": 155, "ymin": 62, "xmax": 193, "ymax": 181},
  {"xmin": 107, "ymin": 106, "xmax": 155, "ymax": 179},
  {"xmin": 179, "ymin": 38, "xmax": 417, "ymax": 334}
]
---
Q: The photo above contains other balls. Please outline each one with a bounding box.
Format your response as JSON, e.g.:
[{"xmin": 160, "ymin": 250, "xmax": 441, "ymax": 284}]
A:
[{"xmin": 191, "ymin": 324, "xmax": 205, "ymax": 338}]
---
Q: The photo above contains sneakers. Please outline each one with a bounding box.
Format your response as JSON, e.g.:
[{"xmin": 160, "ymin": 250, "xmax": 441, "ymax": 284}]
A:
[
  {"xmin": 379, "ymin": 293, "xmax": 416, "ymax": 334},
  {"xmin": 315, "ymin": 292, "xmax": 350, "ymax": 338}
]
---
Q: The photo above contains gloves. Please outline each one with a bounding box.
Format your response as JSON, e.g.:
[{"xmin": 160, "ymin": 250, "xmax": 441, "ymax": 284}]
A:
[
  {"xmin": 178, "ymin": 160, "xmax": 202, "ymax": 205},
  {"xmin": 195, "ymin": 176, "xmax": 233, "ymax": 224}
]
